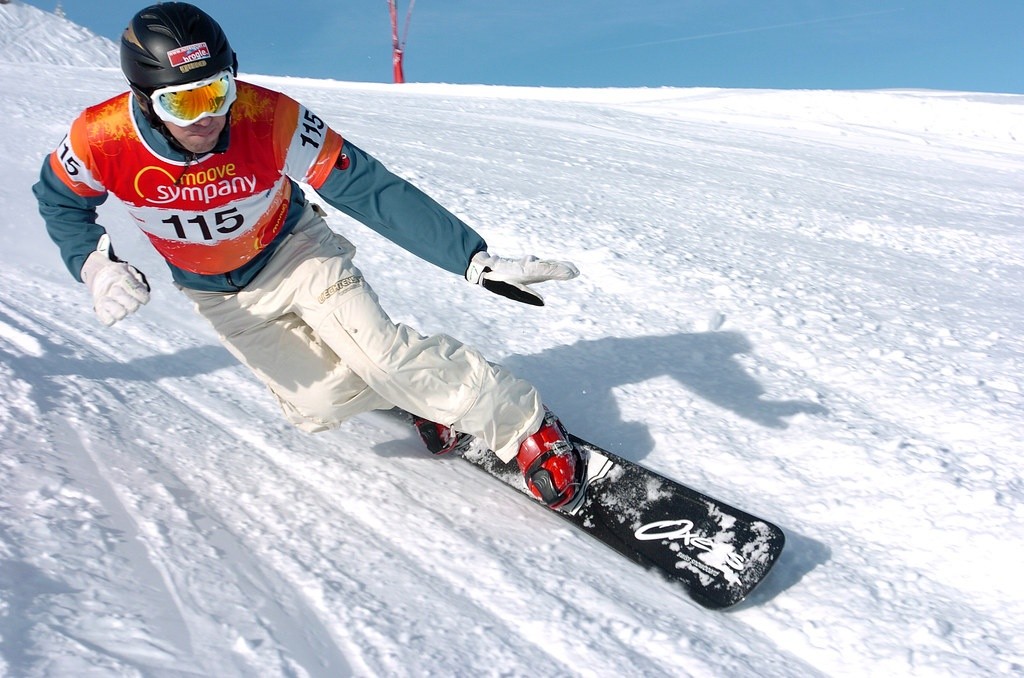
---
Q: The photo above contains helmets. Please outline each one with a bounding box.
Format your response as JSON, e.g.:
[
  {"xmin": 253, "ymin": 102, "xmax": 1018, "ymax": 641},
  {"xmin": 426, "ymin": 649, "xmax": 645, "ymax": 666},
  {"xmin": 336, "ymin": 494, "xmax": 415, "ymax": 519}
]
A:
[{"xmin": 120, "ymin": 2, "xmax": 239, "ymax": 125}]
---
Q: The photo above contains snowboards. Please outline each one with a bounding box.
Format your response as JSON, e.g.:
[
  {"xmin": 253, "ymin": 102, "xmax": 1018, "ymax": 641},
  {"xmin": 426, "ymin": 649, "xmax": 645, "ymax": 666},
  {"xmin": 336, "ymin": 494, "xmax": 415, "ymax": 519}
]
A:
[{"xmin": 455, "ymin": 424, "xmax": 786, "ymax": 612}]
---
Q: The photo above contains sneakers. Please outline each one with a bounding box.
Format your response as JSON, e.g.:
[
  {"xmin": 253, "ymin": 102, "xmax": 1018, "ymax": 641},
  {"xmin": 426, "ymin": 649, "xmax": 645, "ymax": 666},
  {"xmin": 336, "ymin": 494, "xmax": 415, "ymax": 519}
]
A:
[
  {"xmin": 514, "ymin": 406, "xmax": 584, "ymax": 509},
  {"xmin": 408, "ymin": 412, "xmax": 457, "ymax": 455}
]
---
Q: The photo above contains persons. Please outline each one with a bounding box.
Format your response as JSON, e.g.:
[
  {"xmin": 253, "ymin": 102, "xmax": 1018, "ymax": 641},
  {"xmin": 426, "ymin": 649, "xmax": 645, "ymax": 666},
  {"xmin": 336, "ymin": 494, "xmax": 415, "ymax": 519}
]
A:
[{"xmin": 31, "ymin": 2, "xmax": 586, "ymax": 509}]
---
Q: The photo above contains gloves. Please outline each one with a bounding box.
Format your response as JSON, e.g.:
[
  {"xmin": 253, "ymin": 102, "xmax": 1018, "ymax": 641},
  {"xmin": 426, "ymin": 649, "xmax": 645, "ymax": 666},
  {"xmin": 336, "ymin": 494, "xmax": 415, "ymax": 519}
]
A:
[
  {"xmin": 79, "ymin": 233, "xmax": 150, "ymax": 326},
  {"xmin": 464, "ymin": 252, "xmax": 579, "ymax": 306}
]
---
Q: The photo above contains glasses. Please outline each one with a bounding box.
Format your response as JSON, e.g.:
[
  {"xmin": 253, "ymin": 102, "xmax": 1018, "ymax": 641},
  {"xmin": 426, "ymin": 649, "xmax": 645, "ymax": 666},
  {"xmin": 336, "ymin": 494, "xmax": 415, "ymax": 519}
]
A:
[{"xmin": 150, "ymin": 70, "xmax": 238, "ymax": 127}]
